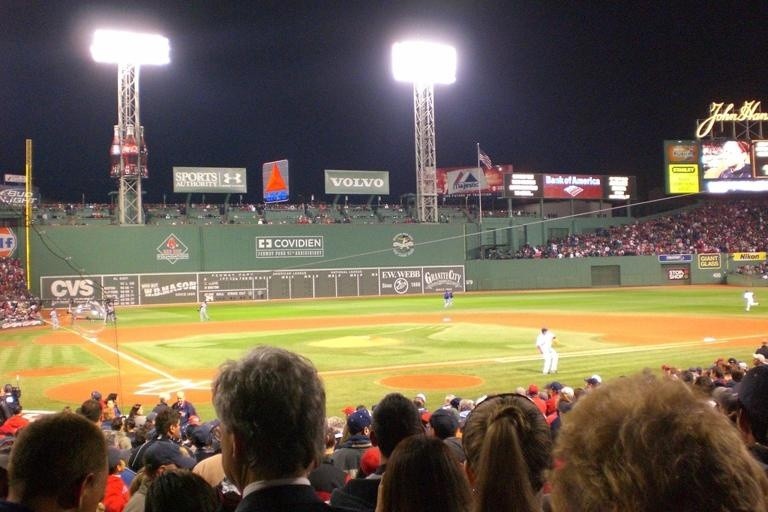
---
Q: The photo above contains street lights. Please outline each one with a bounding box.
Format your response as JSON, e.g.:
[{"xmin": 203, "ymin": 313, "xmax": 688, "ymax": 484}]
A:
[
  {"xmin": 389, "ymin": 38, "xmax": 459, "ymax": 223},
  {"xmin": 88, "ymin": 26, "xmax": 172, "ymax": 226}
]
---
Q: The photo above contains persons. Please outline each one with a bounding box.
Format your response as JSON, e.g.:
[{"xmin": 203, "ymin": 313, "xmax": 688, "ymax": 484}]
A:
[
  {"xmin": 440, "ymin": 290, "xmax": 457, "ymax": 310},
  {"xmin": 536, "ymin": 326, "xmax": 562, "ymax": 377},
  {"xmin": 1, "ymin": 339, "xmax": 768, "ymax": 512},
  {"xmin": 479, "ymin": 194, "xmax": 767, "ymax": 279},
  {"xmin": 742, "ymin": 289, "xmax": 761, "ymax": 314},
  {"xmin": 197, "ymin": 300, "xmax": 212, "ymax": 320},
  {"xmin": 440, "ymin": 204, "xmax": 477, "ymax": 223},
  {"xmin": 718, "ymin": 136, "xmax": 749, "ymax": 180},
  {"xmin": 0, "ymin": 250, "xmax": 59, "ymax": 333},
  {"xmin": 147, "ymin": 201, "xmax": 419, "ymax": 224},
  {"xmin": 33, "ymin": 202, "xmax": 116, "ymax": 222}
]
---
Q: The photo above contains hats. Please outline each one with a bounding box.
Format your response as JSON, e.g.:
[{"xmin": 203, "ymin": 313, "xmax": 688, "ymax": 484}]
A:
[
  {"xmin": 192, "ymin": 425, "xmax": 219, "ymax": 453},
  {"xmin": 347, "ymin": 410, "xmax": 371, "ymax": 433},
  {"xmin": 753, "ymin": 353, "xmax": 765, "ymax": 363},
  {"xmin": 528, "ymin": 375, "xmax": 601, "ymax": 393},
  {"xmin": 360, "ymin": 448, "xmax": 381, "ymax": 474},
  {"xmin": 423, "ymin": 409, "xmax": 457, "ymax": 435},
  {"xmin": 144, "ymin": 442, "xmax": 196, "ymax": 469},
  {"xmin": 109, "ymin": 447, "xmax": 128, "ymax": 470}
]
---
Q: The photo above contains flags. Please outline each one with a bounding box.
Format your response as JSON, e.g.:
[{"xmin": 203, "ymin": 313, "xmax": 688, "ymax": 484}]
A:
[{"xmin": 478, "ymin": 145, "xmax": 495, "ymax": 169}]
